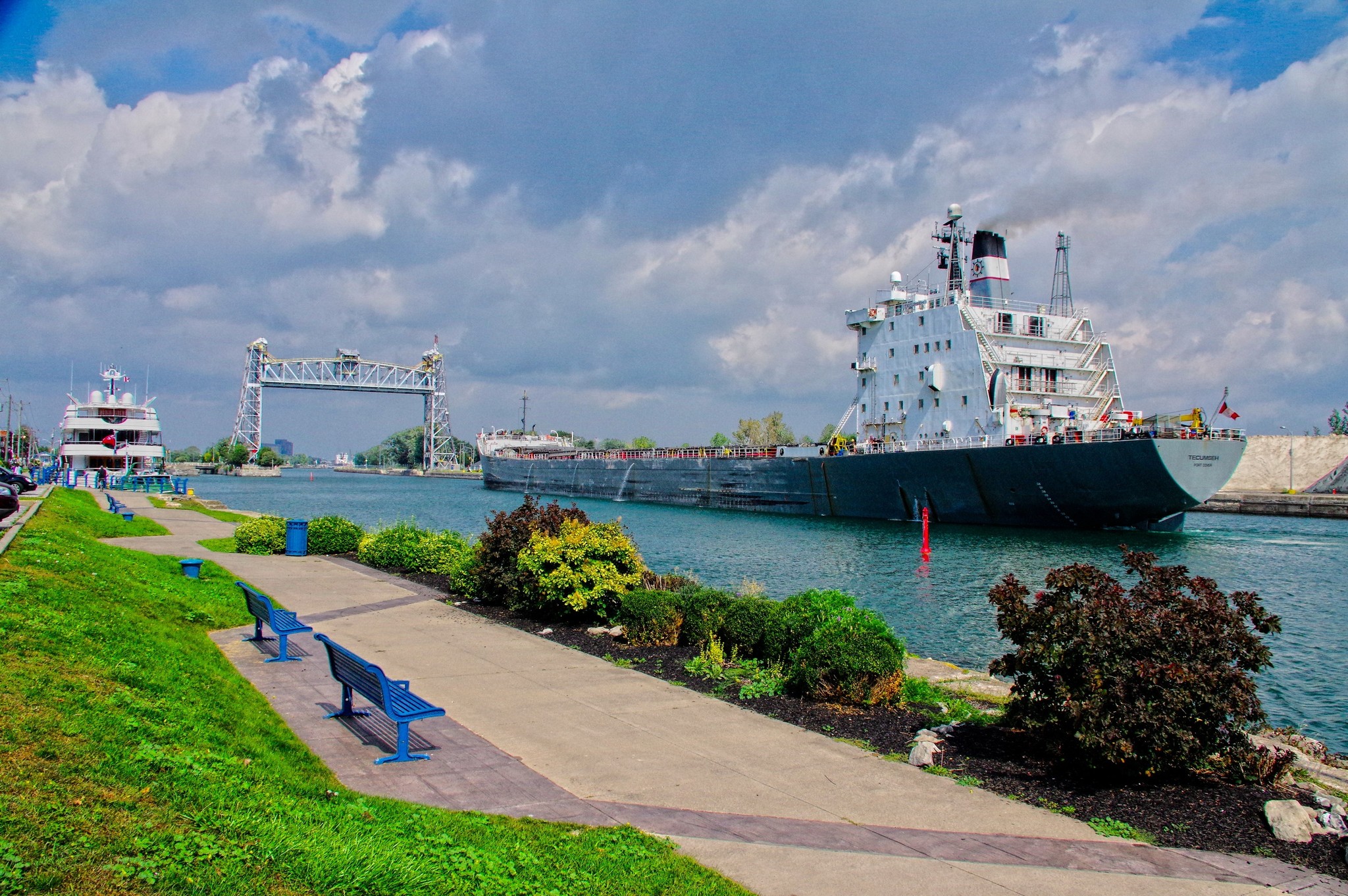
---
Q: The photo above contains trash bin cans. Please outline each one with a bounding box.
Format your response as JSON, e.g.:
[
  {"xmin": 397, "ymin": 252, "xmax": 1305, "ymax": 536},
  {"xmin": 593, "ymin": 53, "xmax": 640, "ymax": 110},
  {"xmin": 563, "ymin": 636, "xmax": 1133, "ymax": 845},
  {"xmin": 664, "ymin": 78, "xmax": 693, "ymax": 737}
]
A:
[{"xmin": 286, "ymin": 519, "xmax": 308, "ymax": 556}]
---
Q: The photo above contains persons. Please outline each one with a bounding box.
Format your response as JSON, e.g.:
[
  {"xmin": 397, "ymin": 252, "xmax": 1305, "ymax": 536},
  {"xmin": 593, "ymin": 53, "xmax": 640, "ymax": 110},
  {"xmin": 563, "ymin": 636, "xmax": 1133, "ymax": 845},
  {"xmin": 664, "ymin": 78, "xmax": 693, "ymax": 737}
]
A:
[
  {"xmin": 12, "ymin": 463, "xmax": 22, "ymax": 475},
  {"xmin": 98, "ymin": 466, "xmax": 107, "ymax": 489},
  {"xmin": 28, "ymin": 462, "xmax": 34, "ymax": 471}
]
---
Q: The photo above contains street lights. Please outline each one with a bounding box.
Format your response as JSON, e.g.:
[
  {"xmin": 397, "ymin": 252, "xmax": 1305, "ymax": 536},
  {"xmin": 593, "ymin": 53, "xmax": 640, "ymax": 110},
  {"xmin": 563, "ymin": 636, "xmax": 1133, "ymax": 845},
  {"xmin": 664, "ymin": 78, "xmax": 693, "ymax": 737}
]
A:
[
  {"xmin": 460, "ymin": 451, "xmax": 465, "ymax": 471},
  {"xmin": 42, "ymin": 439, "xmax": 61, "ymax": 461},
  {"xmin": 1279, "ymin": 426, "xmax": 1292, "ymax": 490},
  {"xmin": 363, "ymin": 455, "xmax": 367, "ymax": 469}
]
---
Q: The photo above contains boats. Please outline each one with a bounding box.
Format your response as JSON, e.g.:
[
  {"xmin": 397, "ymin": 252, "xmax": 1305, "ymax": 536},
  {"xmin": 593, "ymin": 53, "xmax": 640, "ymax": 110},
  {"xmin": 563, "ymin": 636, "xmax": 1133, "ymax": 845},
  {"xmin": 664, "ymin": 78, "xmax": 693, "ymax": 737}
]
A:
[{"xmin": 58, "ymin": 360, "xmax": 173, "ymax": 484}]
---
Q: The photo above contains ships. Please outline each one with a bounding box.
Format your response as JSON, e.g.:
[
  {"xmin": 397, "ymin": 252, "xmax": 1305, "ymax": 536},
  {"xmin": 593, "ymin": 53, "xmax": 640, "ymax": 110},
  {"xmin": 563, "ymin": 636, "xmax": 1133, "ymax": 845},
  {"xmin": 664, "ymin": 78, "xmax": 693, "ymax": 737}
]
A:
[{"xmin": 475, "ymin": 202, "xmax": 1249, "ymax": 549}]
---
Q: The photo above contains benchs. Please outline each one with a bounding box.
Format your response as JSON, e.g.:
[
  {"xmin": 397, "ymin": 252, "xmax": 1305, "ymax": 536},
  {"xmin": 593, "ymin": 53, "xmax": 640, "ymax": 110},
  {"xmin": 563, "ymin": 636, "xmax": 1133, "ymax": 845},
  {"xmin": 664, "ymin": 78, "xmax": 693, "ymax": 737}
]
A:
[
  {"xmin": 314, "ymin": 633, "xmax": 445, "ymax": 766},
  {"xmin": 105, "ymin": 493, "xmax": 135, "ymax": 521},
  {"xmin": 235, "ymin": 580, "xmax": 313, "ymax": 663}
]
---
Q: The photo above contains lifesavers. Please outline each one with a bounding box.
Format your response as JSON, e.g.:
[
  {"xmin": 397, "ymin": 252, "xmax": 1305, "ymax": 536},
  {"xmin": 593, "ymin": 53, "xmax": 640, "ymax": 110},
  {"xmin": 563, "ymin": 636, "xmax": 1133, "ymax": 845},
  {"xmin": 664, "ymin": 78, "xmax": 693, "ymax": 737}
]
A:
[
  {"xmin": 1041, "ymin": 426, "xmax": 1049, "ymax": 435},
  {"xmin": 869, "ymin": 309, "xmax": 877, "ymax": 316}
]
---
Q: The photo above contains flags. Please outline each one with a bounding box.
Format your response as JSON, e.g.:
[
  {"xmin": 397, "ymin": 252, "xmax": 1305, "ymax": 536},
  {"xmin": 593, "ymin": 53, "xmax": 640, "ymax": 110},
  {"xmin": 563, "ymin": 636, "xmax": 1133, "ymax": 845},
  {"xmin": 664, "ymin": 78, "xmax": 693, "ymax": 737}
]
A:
[
  {"xmin": 1219, "ymin": 402, "xmax": 1240, "ymax": 420},
  {"xmin": 124, "ymin": 377, "xmax": 130, "ymax": 382}
]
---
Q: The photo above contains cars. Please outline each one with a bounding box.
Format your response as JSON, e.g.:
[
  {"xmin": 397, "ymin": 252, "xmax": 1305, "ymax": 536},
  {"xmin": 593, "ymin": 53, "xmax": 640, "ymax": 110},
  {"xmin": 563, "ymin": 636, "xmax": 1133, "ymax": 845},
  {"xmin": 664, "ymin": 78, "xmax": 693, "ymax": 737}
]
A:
[
  {"xmin": 11, "ymin": 458, "xmax": 26, "ymax": 464},
  {"xmin": 0, "ymin": 466, "xmax": 38, "ymax": 495},
  {"xmin": 0, "ymin": 482, "xmax": 19, "ymax": 522}
]
